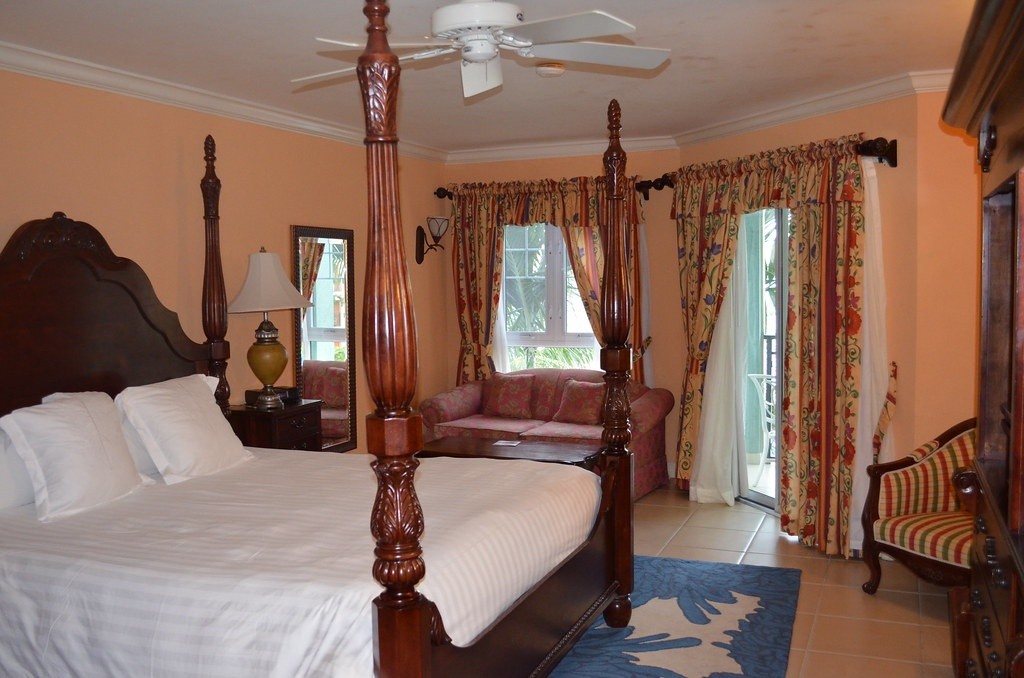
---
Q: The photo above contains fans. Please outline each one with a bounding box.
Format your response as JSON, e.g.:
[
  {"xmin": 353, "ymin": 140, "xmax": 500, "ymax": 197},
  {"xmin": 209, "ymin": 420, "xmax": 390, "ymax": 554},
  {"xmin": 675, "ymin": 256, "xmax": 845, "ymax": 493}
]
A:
[{"xmin": 289, "ymin": 0, "xmax": 672, "ymax": 98}]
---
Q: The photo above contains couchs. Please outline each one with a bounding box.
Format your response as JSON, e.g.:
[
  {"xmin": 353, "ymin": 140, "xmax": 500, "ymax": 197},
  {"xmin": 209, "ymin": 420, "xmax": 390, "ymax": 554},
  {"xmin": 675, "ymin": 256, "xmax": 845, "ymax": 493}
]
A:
[{"xmin": 418, "ymin": 368, "xmax": 675, "ymax": 501}]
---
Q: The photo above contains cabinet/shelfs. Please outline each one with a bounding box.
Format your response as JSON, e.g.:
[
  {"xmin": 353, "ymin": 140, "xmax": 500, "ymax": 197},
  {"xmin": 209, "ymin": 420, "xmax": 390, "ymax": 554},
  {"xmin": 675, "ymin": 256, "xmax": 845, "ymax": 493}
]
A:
[{"xmin": 941, "ymin": 1, "xmax": 1024, "ymax": 678}]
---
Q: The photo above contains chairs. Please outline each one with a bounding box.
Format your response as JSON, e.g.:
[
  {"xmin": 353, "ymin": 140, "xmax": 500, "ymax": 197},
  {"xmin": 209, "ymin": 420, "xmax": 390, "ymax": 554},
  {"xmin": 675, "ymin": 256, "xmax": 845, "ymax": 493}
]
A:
[{"xmin": 861, "ymin": 417, "xmax": 976, "ymax": 595}]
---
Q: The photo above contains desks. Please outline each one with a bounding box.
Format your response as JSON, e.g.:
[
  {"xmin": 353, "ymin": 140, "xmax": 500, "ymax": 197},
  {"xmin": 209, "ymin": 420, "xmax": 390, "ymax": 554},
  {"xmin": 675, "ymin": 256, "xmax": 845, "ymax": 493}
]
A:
[{"xmin": 423, "ymin": 437, "xmax": 603, "ymax": 470}]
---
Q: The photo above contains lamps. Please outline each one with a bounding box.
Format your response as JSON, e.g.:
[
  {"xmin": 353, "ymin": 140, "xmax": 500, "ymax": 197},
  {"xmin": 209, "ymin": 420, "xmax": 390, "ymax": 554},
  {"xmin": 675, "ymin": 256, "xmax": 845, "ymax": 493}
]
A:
[
  {"xmin": 416, "ymin": 217, "xmax": 450, "ymax": 264},
  {"xmin": 226, "ymin": 245, "xmax": 315, "ymax": 414}
]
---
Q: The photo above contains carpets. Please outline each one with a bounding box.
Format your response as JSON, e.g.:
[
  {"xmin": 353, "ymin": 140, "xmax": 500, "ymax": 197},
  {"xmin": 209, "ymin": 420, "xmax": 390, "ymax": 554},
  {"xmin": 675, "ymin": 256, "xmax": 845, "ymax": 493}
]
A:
[{"xmin": 547, "ymin": 554, "xmax": 802, "ymax": 678}]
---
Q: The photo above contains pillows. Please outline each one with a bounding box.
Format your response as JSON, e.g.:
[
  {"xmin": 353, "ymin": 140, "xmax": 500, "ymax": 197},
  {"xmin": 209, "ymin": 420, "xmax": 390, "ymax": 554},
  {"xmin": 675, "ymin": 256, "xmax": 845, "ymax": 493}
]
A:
[
  {"xmin": 483, "ymin": 372, "xmax": 535, "ymax": 419},
  {"xmin": 552, "ymin": 377, "xmax": 609, "ymax": 425},
  {"xmin": 0, "ymin": 374, "xmax": 257, "ymax": 524}
]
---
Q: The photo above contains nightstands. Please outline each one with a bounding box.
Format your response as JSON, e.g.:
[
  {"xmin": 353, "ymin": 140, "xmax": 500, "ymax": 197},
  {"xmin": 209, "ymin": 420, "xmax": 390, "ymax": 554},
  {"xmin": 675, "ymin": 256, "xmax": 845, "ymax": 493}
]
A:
[{"xmin": 225, "ymin": 399, "xmax": 324, "ymax": 452}]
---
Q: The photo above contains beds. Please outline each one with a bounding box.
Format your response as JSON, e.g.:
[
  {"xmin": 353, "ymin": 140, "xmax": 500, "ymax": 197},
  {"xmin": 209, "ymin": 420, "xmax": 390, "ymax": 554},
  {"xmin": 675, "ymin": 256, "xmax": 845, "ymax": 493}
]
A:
[{"xmin": 0, "ymin": 0, "xmax": 636, "ymax": 678}]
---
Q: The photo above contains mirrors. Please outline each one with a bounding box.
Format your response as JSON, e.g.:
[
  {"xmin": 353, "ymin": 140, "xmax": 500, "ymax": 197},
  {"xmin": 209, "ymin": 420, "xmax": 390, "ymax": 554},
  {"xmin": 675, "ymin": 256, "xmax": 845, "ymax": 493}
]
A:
[{"xmin": 290, "ymin": 225, "xmax": 358, "ymax": 454}]
